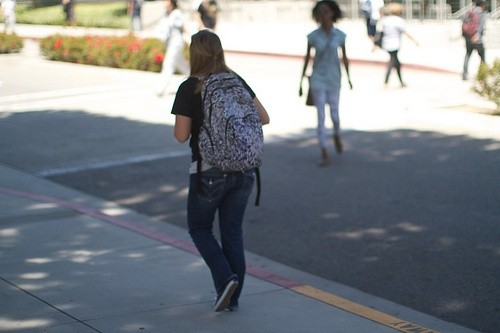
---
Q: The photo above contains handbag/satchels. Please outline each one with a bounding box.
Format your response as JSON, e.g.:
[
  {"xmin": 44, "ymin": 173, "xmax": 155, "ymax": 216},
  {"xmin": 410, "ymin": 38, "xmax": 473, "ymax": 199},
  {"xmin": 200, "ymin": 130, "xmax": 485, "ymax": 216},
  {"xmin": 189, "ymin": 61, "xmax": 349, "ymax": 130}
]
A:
[{"xmin": 305, "ymin": 76, "xmax": 317, "ymax": 106}]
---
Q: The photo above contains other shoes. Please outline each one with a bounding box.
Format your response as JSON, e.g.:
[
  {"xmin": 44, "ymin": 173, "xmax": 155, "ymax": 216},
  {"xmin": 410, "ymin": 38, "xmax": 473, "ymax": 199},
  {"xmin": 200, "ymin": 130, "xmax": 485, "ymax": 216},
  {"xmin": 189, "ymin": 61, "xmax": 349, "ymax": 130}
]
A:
[
  {"xmin": 335, "ymin": 136, "xmax": 343, "ymax": 152},
  {"xmin": 321, "ymin": 157, "xmax": 330, "ymax": 165}
]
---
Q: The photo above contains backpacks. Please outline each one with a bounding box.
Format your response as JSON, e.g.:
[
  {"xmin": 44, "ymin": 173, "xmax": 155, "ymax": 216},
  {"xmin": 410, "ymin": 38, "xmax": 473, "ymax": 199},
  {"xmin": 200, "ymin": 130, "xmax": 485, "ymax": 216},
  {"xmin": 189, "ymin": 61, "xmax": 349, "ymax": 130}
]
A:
[
  {"xmin": 186, "ymin": 69, "xmax": 264, "ymax": 173},
  {"xmin": 462, "ymin": 6, "xmax": 483, "ymax": 37}
]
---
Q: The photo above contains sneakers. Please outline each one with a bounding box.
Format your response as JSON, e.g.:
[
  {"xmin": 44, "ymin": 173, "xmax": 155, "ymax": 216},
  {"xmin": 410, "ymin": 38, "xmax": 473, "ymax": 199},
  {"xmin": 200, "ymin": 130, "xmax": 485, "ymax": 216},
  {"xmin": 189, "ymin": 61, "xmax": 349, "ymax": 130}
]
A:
[
  {"xmin": 213, "ymin": 277, "xmax": 239, "ymax": 313},
  {"xmin": 224, "ymin": 304, "xmax": 239, "ymax": 312}
]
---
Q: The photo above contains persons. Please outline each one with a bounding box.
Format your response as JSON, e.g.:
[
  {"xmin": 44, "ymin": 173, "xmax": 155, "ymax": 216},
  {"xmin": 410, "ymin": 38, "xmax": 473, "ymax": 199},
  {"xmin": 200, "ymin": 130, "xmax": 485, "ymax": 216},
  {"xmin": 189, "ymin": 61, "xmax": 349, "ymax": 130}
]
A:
[
  {"xmin": 461, "ymin": 0, "xmax": 486, "ymax": 80},
  {"xmin": 359, "ymin": 0, "xmax": 420, "ymax": 88},
  {"xmin": 129, "ymin": 0, "xmax": 144, "ymax": 32},
  {"xmin": 62, "ymin": 0, "xmax": 77, "ymax": 27},
  {"xmin": 153, "ymin": 0, "xmax": 189, "ymax": 97},
  {"xmin": 0, "ymin": 0, "xmax": 16, "ymax": 34},
  {"xmin": 299, "ymin": 0, "xmax": 353, "ymax": 166},
  {"xmin": 170, "ymin": 29, "xmax": 271, "ymax": 312},
  {"xmin": 194, "ymin": 0, "xmax": 218, "ymax": 32}
]
